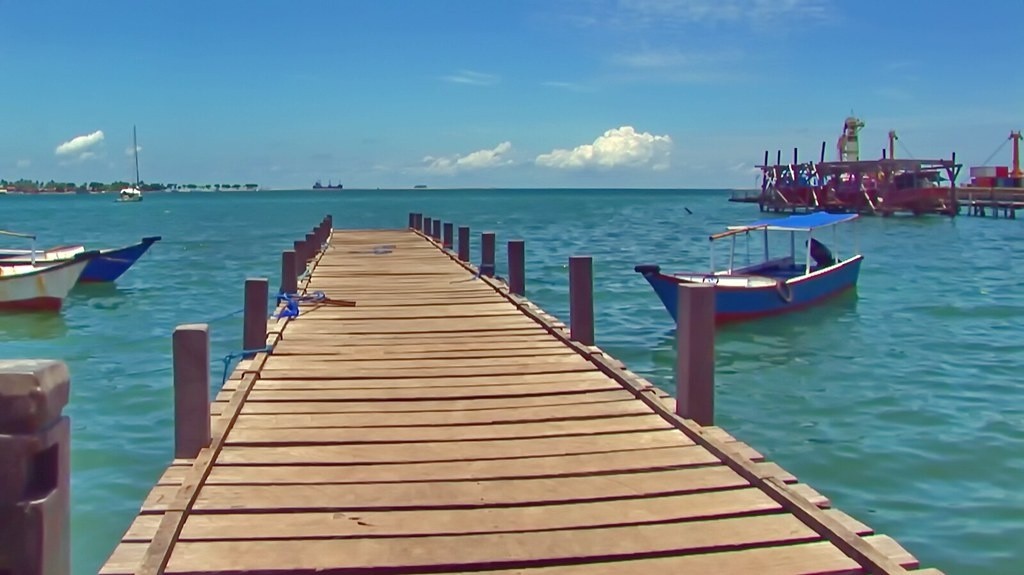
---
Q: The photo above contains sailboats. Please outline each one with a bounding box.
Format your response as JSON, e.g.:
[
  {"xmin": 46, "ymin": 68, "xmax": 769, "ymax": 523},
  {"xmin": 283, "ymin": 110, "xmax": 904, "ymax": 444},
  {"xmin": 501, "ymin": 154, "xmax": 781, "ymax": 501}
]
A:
[{"xmin": 115, "ymin": 124, "xmax": 143, "ymax": 202}]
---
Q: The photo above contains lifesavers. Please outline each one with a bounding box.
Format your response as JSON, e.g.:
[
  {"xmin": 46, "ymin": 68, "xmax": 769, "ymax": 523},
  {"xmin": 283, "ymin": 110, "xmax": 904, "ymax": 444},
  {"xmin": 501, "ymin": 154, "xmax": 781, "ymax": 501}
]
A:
[
  {"xmin": 633, "ymin": 264, "xmax": 661, "ymax": 273},
  {"xmin": 776, "ymin": 279, "xmax": 794, "ymax": 302},
  {"xmin": 74, "ymin": 251, "xmax": 103, "ymax": 260},
  {"xmin": 142, "ymin": 235, "xmax": 162, "ymax": 243}
]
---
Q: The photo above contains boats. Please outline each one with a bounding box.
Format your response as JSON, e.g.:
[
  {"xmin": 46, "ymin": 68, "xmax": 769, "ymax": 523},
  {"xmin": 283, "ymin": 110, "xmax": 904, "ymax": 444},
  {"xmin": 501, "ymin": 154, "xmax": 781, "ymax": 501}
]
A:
[
  {"xmin": 634, "ymin": 212, "xmax": 864, "ymax": 327},
  {"xmin": 0, "ymin": 230, "xmax": 162, "ymax": 310},
  {"xmin": 312, "ymin": 178, "xmax": 344, "ymax": 189}
]
